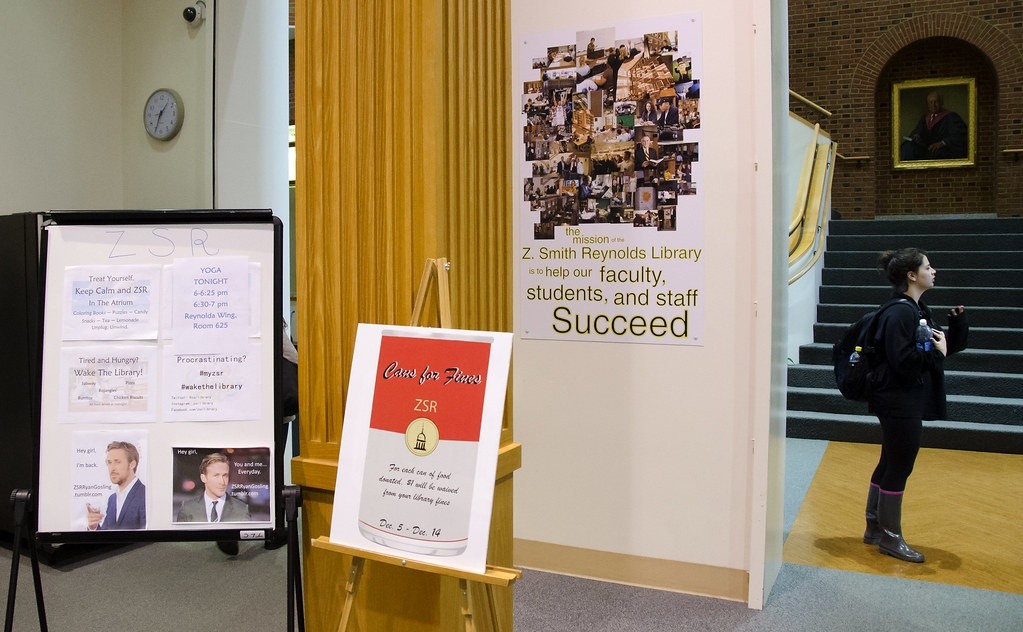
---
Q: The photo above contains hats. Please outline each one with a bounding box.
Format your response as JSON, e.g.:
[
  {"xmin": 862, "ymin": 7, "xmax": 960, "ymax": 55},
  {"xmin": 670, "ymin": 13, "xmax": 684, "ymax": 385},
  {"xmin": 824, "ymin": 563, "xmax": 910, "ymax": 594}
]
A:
[{"xmin": 655, "ymin": 98, "xmax": 669, "ymax": 106}]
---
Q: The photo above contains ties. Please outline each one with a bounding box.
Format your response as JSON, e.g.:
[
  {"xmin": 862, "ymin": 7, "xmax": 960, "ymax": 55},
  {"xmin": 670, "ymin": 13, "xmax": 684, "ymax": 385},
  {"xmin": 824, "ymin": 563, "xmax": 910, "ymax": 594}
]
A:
[{"xmin": 211, "ymin": 501, "xmax": 219, "ymax": 522}]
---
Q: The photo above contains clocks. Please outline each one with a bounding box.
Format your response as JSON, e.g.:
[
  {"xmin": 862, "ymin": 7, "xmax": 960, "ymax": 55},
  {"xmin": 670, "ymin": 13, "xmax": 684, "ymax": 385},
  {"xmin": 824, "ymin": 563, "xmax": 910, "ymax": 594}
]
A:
[{"xmin": 143, "ymin": 88, "xmax": 185, "ymax": 141}]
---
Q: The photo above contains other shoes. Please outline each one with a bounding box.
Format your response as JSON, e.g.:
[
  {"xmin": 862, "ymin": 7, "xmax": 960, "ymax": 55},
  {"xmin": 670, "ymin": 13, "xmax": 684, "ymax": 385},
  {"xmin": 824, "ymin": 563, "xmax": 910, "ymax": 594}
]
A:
[
  {"xmin": 217, "ymin": 540, "xmax": 239, "ymax": 555},
  {"xmin": 264, "ymin": 539, "xmax": 286, "ymax": 550}
]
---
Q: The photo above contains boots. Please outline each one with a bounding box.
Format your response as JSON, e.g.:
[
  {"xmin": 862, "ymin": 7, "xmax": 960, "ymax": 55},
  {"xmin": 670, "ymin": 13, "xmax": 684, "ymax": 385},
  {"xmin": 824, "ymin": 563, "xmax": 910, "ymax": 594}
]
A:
[
  {"xmin": 878, "ymin": 487, "xmax": 925, "ymax": 562},
  {"xmin": 862, "ymin": 482, "xmax": 882, "ymax": 544}
]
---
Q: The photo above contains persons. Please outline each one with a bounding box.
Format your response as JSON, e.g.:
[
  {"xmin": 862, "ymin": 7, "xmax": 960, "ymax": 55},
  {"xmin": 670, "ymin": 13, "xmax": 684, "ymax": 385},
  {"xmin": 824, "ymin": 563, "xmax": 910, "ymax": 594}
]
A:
[
  {"xmin": 177, "ymin": 453, "xmax": 250, "ymax": 522},
  {"xmin": 862, "ymin": 246, "xmax": 970, "ymax": 563},
  {"xmin": 524, "ymin": 37, "xmax": 700, "ymax": 239},
  {"xmin": 86, "ymin": 441, "xmax": 146, "ymax": 530}
]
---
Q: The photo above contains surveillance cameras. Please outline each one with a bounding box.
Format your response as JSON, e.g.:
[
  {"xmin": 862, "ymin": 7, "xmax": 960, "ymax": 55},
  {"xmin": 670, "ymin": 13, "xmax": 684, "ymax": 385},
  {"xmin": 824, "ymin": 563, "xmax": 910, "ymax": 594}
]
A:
[{"xmin": 182, "ymin": 4, "xmax": 202, "ymax": 26}]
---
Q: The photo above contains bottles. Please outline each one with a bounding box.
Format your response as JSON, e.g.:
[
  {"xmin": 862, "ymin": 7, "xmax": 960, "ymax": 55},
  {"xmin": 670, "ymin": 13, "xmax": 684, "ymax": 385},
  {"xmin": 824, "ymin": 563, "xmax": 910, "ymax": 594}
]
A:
[
  {"xmin": 850, "ymin": 346, "xmax": 863, "ymax": 377},
  {"xmin": 915, "ymin": 319, "xmax": 934, "ymax": 353}
]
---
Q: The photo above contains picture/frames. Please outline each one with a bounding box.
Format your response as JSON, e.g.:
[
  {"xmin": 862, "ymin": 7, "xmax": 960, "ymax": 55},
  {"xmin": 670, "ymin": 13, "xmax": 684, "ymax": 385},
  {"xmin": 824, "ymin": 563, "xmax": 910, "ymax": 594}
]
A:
[{"xmin": 891, "ymin": 76, "xmax": 978, "ymax": 170}]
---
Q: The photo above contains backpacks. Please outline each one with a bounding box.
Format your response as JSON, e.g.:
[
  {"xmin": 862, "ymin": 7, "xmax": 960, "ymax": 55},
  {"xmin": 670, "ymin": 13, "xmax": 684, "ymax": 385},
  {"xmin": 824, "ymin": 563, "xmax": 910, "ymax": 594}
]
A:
[{"xmin": 832, "ymin": 298, "xmax": 920, "ymax": 401}]
